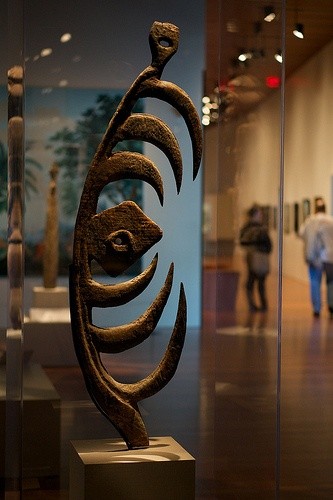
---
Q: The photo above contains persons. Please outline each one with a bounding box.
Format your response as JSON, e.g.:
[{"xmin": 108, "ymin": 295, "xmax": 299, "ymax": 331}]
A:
[
  {"xmin": 238, "ymin": 204, "xmax": 273, "ymax": 314},
  {"xmin": 298, "ymin": 199, "xmax": 333, "ymax": 318}
]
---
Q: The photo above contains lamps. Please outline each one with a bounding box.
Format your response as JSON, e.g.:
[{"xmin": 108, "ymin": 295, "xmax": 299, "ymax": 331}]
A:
[
  {"xmin": 292, "ymin": 24, "xmax": 304, "ymax": 39},
  {"xmin": 263, "ymin": 6, "xmax": 275, "ymax": 23},
  {"xmin": 238, "ymin": 47, "xmax": 246, "ymax": 62},
  {"xmin": 274, "ymin": 48, "xmax": 283, "ymax": 64}
]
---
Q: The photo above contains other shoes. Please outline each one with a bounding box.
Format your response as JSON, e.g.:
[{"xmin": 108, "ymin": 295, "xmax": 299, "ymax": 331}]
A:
[
  {"xmin": 329, "ymin": 307, "xmax": 333, "ymax": 313},
  {"xmin": 314, "ymin": 311, "xmax": 319, "ymax": 316},
  {"xmin": 250, "ymin": 304, "xmax": 268, "ymax": 312}
]
